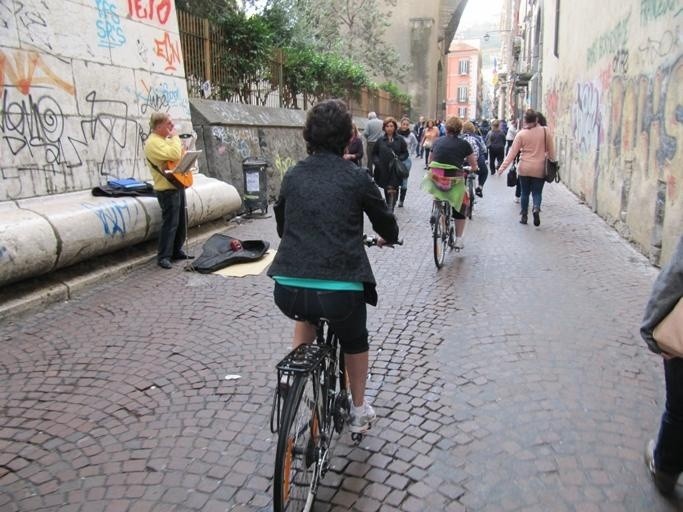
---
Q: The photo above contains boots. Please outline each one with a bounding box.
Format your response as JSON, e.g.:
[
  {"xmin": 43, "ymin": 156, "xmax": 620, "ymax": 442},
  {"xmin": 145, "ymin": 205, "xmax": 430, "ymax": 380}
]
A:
[
  {"xmin": 387, "ymin": 191, "xmax": 397, "ymax": 213},
  {"xmin": 399, "ymin": 189, "xmax": 406, "ymax": 208}
]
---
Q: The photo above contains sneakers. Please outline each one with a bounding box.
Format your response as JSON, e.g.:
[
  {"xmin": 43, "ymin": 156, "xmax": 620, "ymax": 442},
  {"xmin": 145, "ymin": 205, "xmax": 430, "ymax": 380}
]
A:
[
  {"xmin": 349, "ymin": 401, "xmax": 376, "ymax": 433},
  {"xmin": 452, "ymin": 239, "xmax": 465, "ymax": 250},
  {"xmin": 645, "ymin": 437, "xmax": 679, "ymax": 497},
  {"xmin": 514, "ymin": 195, "xmax": 520, "ymax": 202},
  {"xmin": 276, "ymin": 363, "xmax": 295, "ymax": 398},
  {"xmin": 430, "ymin": 210, "xmax": 439, "ymax": 224},
  {"xmin": 475, "ymin": 187, "xmax": 484, "ymax": 198},
  {"xmin": 491, "ymin": 172, "xmax": 496, "ymax": 174}
]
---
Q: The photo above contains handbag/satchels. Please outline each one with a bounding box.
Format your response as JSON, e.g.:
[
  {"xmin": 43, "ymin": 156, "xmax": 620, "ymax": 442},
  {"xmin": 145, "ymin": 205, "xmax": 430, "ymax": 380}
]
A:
[
  {"xmin": 543, "ymin": 126, "xmax": 559, "ymax": 182},
  {"xmin": 506, "ymin": 157, "xmax": 518, "ymax": 187},
  {"xmin": 652, "ymin": 294, "xmax": 682, "ymax": 359},
  {"xmin": 384, "ymin": 137, "xmax": 408, "ymax": 180},
  {"xmin": 486, "ymin": 129, "xmax": 493, "ymax": 149}
]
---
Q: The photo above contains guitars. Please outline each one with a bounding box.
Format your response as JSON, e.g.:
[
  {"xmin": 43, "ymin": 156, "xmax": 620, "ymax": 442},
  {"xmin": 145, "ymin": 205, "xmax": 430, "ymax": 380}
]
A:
[{"xmin": 162, "ymin": 134, "xmax": 193, "ymax": 190}]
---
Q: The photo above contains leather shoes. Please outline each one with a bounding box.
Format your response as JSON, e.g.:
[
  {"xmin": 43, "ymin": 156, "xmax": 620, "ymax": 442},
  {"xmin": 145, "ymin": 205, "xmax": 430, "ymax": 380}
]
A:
[
  {"xmin": 175, "ymin": 253, "xmax": 196, "ymax": 260},
  {"xmin": 533, "ymin": 206, "xmax": 542, "ymax": 227},
  {"xmin": 425, "ymin": 167, "xmax": 426, "ymax": 169},
  {"xmin": 519, "ymin": 214, "xmax": 528, "ymax": 224},
  {"xmin": 156, "ymin": 257, "xmax": 174, "ymax": 269},
  {"xmin": 427, "ymin": 168, "xmax": 429, "ymax": 169}
]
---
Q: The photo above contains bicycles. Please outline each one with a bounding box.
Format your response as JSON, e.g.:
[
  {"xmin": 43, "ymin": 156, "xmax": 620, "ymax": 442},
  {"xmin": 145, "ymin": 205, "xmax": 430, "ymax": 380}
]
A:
[
  {"xmin": 464, "ymin": 146, "xmax": 489, "ymax": 220},
  {"xmin": 422, "ymin": 165, "xmax": 480, "ymax": 269},
  {"xmin": 267, "ymin": 233, "xmax": 405, "ymax": 512}
]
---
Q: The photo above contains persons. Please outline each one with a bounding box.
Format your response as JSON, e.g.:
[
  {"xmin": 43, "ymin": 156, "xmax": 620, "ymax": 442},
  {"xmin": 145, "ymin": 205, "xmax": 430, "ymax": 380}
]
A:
[
  {"xmin": 484, "ymin": 121, "xmax": 507, "ymax": 175},
  {"xmin": 420, "ymin": 119, "xmax": 440, "ymax": 169},
  {"xmin": 370, "ymin": 118, "xmax": 409, "ymax": 212},
  {"xmin": 413, "ymin": 115, "xmax": 427, "ymax": 158},
  {"xmin": 458, "ymin": 123, "xmax": 489, "ymax": 197},
  {"xmin": 142, "ymin": 114, "xmax": 197, "ymax": 269},
  {"xmin": 507, "ymin": 118, "xmax": 520, "ymax": 151},
  {"xmin": 495, "ymin": 117, "xmax": 514, "ymax": 133},
  {"xmin": 427, "ymin": 116, "xmax": 479, "ymax": 250},
  {"xmin": 266, "ymin": 100, "xmax": 400, "ymax": 434},
  {"xmin": 342, "ymin": 124, "xmax": 365, "ymax": 167},
  {"xmin": 435, "ymin": 117, "xmax": 491, "ymax": 135},
  {"xmin": 496, "ymin": 110, "xmax": 557, "ymax": 227},
  {"xmin": 361, "ymin": 111, "xmax": 385, "ymax": 175},
  {"xmin": 397, "ymin": 116, "xmax": 418, "ymax": 207},
  {"xmin": 640, "ymin": 226, "xmax": 683, "ymax": 497}
]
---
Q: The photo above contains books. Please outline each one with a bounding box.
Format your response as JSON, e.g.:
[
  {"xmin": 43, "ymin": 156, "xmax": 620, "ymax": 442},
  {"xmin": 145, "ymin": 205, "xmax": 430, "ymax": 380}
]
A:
[{"xmin": 165, "ymin": 150, "xmax": 201, "ymax": 174}]
